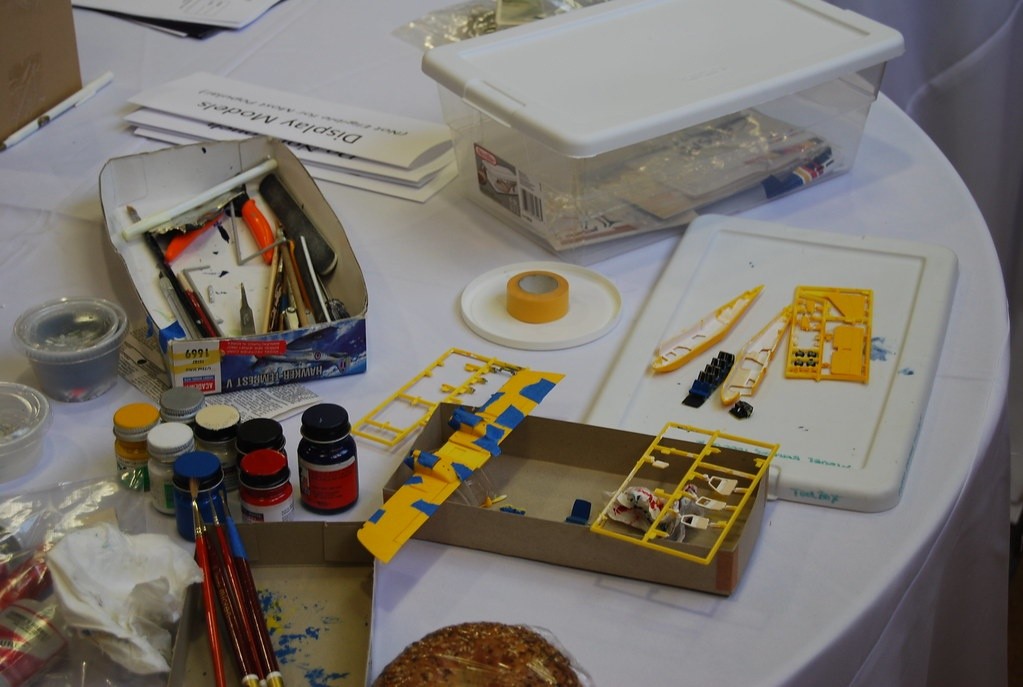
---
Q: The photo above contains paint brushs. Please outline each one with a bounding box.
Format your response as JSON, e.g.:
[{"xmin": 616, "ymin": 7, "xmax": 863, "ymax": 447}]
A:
[
  {"xmin": 190, "ymin": 479, "xmax": 284, "ymax": 687},
  {"xmin": 127, "ymin": 206, "xmax": 217, "ymax": 340}
]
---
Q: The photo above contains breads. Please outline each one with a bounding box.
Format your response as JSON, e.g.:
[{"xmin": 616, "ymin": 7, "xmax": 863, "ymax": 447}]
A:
[{"xmin": 369, "ymin": 620, "xmax": 587, "ymax": 687}]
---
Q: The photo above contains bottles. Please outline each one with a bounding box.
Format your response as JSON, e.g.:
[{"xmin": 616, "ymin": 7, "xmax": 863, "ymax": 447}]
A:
[{"xmin": 111, "ymin": 385, "xmax": 356, "ymax": 540}]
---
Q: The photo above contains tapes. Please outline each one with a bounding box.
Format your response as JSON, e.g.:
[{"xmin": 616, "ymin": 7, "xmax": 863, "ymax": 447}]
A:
[{"xmin": 506, "ymin": 269, "xmax": 569, "ymax": 325}]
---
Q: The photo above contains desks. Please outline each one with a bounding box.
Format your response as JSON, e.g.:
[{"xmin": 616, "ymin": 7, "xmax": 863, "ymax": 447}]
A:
[{"xmin": 1, "ymin": 1, "xmax": 1010, "ymax": 687}]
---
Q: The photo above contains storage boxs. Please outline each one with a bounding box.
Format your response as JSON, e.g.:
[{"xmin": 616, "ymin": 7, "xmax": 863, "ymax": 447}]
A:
[
  {"xmin": 13, "ymin": 295, "xmax": 129, "ymax": 404},
  {"xmin": 420, "ymin": 0, "xmax": 907, "ymax": 267},
  {"xmin": 97, "ymin": 133, "xmax": 369, "ymax": 395},
  {"xmin": 1, "ymin": 379, "xmax": 50, "ymax": 483},
  {"xmin": 1, "ymin": 0, "xmax": 82, "ymax": 152},
  {"xmin": 384, "ymin": 401, "xmax": 767, "ymax": 597},
  {"xmin": 164, "ymin": 519, "xmax": 378, "ymax": 687}
]
